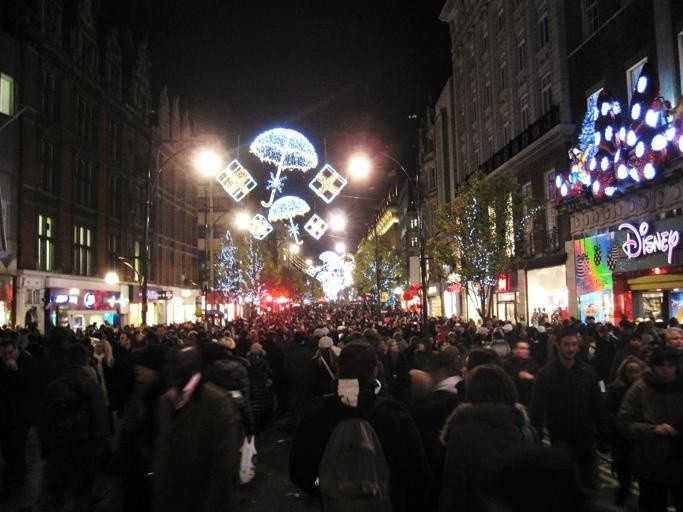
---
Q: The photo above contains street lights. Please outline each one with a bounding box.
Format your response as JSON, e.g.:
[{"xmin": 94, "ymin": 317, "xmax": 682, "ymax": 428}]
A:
[
  {"xmin": 329, "ymin": 206, "xmax": 403, "ymax": 315},
  {"xmin": 105, "ymin": 139, "xmax": 250, "ymax": 325},
  {"xmin": 347, "ymin": 147, "xmax": 429, "ymax": 343}
]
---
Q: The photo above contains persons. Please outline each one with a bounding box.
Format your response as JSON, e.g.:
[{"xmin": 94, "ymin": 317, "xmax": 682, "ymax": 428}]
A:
[{"xmin": 0, "ymin": 297, "xmax": 682, "ymax": 512}]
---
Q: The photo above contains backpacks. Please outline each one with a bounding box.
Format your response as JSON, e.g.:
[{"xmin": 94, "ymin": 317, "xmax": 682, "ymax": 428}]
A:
[{"xmin": 319, "ymin": 417, "xmax": 391, "ymax": 511}]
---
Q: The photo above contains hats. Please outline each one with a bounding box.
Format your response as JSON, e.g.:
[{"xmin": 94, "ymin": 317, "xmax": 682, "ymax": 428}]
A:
[{"xmin": 213, "ymin": 326, "xmax": 381, "ymax": 356}]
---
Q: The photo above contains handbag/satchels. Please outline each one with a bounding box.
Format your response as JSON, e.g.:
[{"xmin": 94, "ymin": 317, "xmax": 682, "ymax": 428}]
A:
[{"xmin": 238, "ymin": 435, "xmax": 259, "ymax": 484}]
